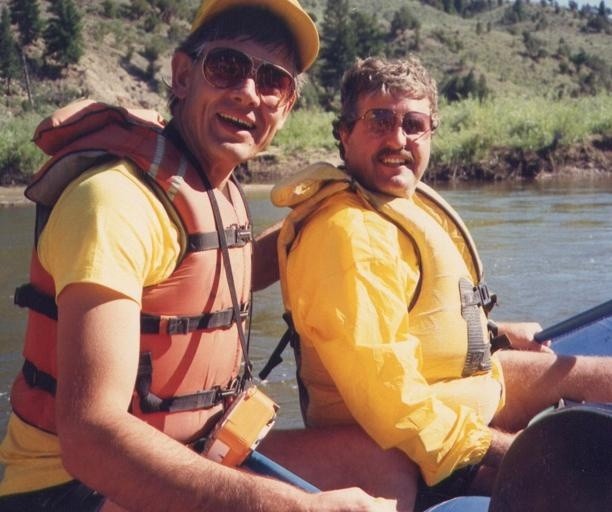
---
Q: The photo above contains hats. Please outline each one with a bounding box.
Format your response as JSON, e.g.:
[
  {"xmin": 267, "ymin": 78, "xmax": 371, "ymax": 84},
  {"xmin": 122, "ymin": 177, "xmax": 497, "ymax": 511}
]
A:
[{"xmin": 193, "ymin": 0, "xmax": 321, "ymax": 73}]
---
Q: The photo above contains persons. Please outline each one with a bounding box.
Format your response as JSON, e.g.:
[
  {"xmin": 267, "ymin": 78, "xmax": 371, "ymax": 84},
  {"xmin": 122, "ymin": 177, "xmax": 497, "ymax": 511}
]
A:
[
  {"xmin": 269, "ymin": 51, "xmax": 612, "ymax": 502},
  {"xmin": 1, "ymin": 0, "xmax": 420, "ymax": 512}
]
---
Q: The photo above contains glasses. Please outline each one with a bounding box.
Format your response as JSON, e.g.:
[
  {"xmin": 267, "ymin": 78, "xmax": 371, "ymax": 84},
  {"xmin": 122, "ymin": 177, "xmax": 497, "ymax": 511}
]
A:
[
  {"xmin": 352, "ymin": 107, "xmax": 434, "ymax": 138},
  {"xmin": 200, "ymin": 46, "xmax": 297, "ymax": 111}
]
